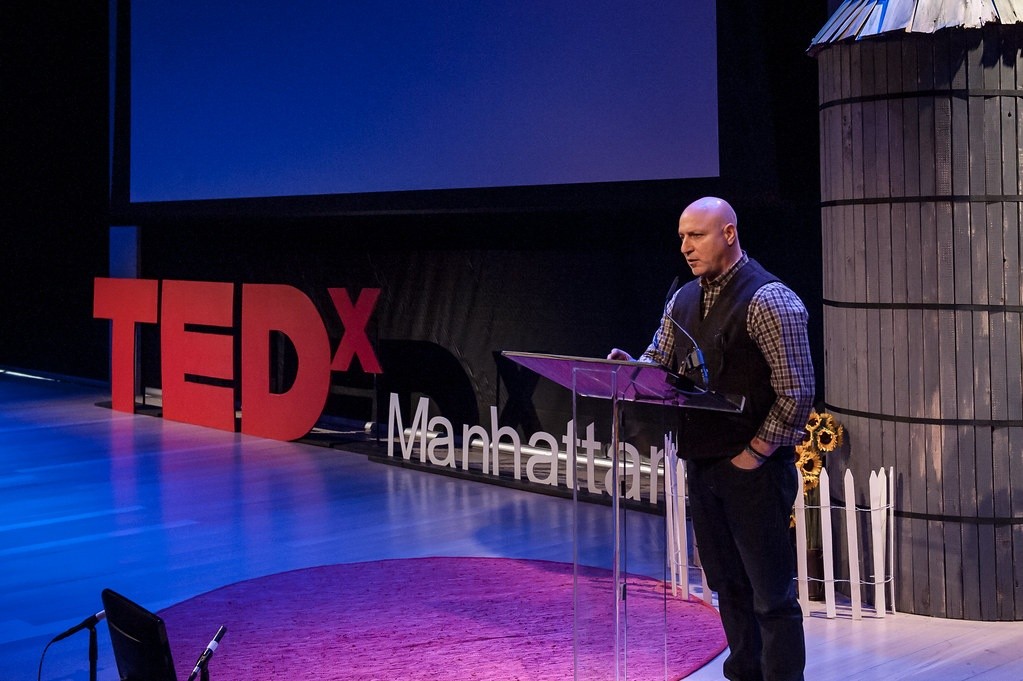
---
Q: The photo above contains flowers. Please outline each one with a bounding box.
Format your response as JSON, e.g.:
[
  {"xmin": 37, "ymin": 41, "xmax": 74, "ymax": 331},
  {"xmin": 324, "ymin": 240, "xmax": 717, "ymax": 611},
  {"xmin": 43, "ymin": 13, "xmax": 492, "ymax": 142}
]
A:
[{"xmin": 789, "ymin": 411, "xmax": 844, "ymax": 530}]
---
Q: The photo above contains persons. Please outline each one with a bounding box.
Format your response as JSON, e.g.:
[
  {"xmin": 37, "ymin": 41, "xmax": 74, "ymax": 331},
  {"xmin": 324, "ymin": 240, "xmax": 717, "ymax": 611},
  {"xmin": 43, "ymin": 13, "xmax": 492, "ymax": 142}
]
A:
[{"xmin": 608, "ymin": 197, "xmax": 814, "ymax": 681}]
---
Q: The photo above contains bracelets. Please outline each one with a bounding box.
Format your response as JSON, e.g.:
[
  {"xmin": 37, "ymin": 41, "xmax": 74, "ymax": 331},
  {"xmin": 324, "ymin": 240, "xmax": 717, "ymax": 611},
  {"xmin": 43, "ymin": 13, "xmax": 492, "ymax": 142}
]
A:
[{"xmin": 745, "ymin": 443, "xmax": 770, "ymax": 464}]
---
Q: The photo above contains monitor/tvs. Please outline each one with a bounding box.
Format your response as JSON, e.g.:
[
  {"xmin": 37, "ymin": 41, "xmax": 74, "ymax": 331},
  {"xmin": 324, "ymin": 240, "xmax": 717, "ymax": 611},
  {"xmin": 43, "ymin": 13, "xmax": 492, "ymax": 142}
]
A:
[{"xmin": 102, "ymin": 588, "xmax": 178, "ymax": 681}]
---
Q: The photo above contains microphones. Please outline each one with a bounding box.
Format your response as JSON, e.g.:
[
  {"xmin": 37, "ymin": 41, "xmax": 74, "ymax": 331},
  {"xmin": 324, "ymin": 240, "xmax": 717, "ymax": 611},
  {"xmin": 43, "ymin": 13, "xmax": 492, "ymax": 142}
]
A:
[
  {"xmin": 664, "ymin": 275, "xmax": 709, "ymax": 388},
  {"xmin": 53, "ymin": 610, "xmax": 106, "ymax": 642},
  {"xmin": 187, "ymin": 625, "xmax": 228, "ymax": 681}
]
